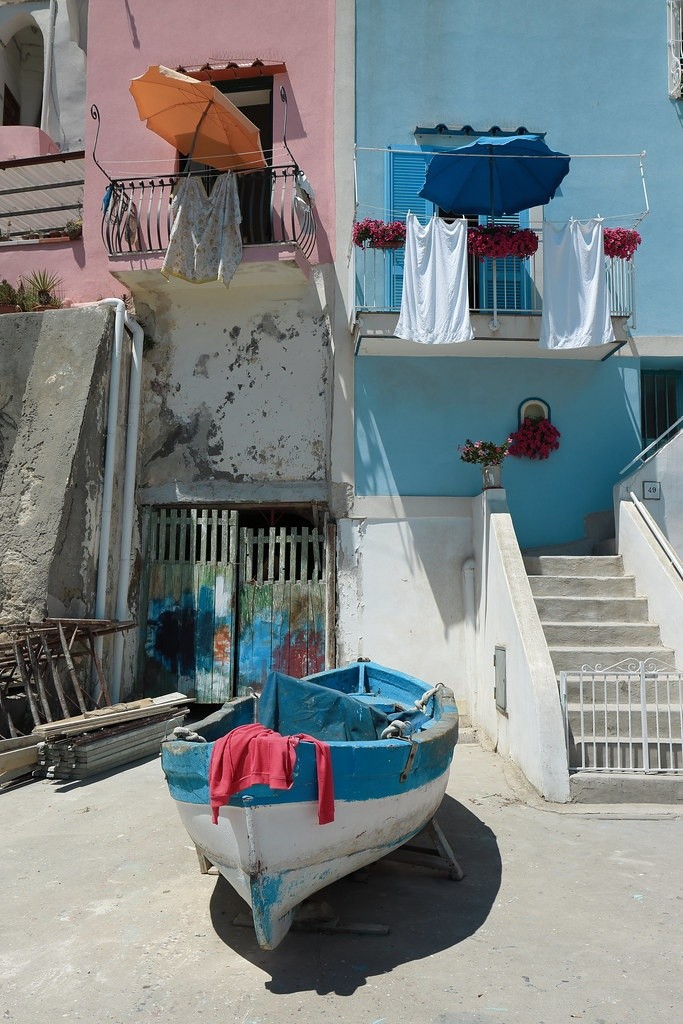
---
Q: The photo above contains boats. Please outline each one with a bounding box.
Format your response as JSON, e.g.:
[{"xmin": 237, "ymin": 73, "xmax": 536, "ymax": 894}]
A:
[{"xmin": 160, "ymin": 656, "xmax": 462, "ymax": 949}]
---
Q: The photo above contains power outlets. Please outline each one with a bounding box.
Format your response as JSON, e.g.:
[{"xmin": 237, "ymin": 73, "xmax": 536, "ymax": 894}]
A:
[{"xmin": 644, "ymin": 481, "xmax": 660, "ymax": 500}]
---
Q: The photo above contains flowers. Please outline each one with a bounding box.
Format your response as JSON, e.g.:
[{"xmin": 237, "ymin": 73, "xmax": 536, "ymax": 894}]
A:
[
  {"xmin": 603, "ymin": 228, "xmax": 643, "ymax": 259},
  {"xmin": 461, "ymin": 416, "xmax": 560, "ymax": 465},
  {"xmin": 468, "ymin": 224, "xmax": 539, "ymax": 261},
  {"xmin": 353, "ymin": 219, "xmax": 406, "ymax": 248}
]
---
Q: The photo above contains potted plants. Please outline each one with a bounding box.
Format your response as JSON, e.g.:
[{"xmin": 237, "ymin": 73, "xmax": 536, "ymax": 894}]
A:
[
  {"xmin": 25, "ymin": 270, "xmax": 60, "ymax": 310},
  {"xmin": 0, "ymin": 279, "xmax": 23, "ymax": 315}
]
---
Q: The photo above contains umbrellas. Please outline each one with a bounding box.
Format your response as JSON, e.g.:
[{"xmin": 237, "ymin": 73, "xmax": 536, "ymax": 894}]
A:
[
  {"xmin": 129, "ymin": 65, "xmax": 268, "ymax": 198},
  {"xmin": 415, "ymin": 134, "xmax": 571, "ymax": 326}
]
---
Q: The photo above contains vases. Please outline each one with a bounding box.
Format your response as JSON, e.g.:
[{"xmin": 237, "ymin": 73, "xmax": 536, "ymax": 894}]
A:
[
  {"xmin": 482, "ymin": 464, "xmax": 502, "ymax": 489},
  {"xmin": 368, "ymin": 238, "xmax": 405, "ymax": 248}
]
